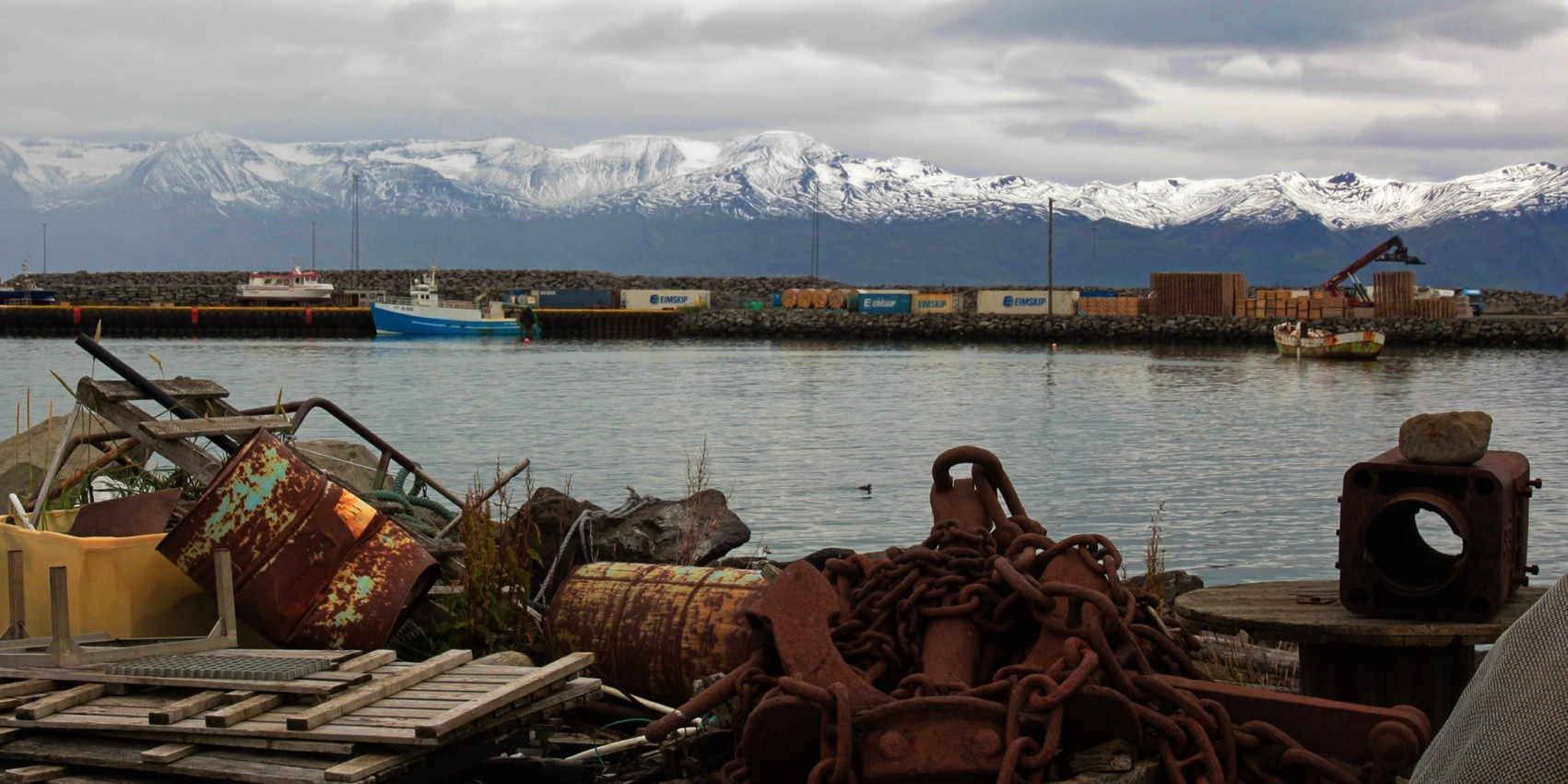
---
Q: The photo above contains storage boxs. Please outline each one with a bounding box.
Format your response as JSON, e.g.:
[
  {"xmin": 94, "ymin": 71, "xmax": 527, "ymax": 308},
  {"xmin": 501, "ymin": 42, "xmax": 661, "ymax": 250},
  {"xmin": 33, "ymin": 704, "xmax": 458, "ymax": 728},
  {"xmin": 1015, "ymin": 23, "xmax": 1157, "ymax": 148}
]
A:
[
  {"xmin": 0, "ymin": 510, "xmax": 213, "ymax": 642},
  {"xmin": 535, "ymin": 272, "xmax": 1476, "ymax": 319}
]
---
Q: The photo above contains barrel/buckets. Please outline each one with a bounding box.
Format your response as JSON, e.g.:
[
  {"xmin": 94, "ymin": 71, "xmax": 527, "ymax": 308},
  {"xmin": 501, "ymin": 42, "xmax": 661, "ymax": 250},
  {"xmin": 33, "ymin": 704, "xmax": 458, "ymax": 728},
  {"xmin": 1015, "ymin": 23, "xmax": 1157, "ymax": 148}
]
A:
[
  {"xmin": 154, "ymin": 426, "xmax": 440, "ymax": 653},
  {"xmin": 540, "ymin": 560, "xmax": 772, "ymax": 708}
]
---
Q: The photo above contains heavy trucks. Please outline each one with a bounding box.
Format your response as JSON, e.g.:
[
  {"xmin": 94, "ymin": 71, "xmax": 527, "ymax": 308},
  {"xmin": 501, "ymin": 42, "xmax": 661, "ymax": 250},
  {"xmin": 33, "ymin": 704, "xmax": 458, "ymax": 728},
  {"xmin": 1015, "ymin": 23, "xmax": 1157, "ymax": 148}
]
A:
[{"xmin": 1357, "ymin": 287, "xmax": 1484, "ymax": 318}]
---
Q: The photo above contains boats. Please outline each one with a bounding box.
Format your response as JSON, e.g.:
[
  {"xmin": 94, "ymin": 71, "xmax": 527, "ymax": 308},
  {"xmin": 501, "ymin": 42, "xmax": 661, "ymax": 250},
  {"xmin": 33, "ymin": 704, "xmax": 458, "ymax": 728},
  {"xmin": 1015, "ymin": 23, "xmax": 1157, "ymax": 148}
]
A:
[
  {"xmin": 234, "ymin": 270, "xmax": 334, "ymax": 304},
  {"xmin": 369, "ymin": 264, "xmax": 539, "ymax": 337},
  {"xmin": 1273, "ymin": 322, "xmax": 1386, "ymax": 360},
  {"xmin": 0, "ymin": 262, "xmax": 58, "ymax": 305}
]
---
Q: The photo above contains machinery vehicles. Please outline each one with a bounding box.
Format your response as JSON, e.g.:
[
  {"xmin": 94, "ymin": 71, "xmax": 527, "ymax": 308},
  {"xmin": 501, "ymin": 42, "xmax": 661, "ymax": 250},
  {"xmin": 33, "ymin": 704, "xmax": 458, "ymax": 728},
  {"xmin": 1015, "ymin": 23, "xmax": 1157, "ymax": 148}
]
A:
[{"xmin": 1317, "ymin": 235, "xmax": 1425, "ymax": 308}]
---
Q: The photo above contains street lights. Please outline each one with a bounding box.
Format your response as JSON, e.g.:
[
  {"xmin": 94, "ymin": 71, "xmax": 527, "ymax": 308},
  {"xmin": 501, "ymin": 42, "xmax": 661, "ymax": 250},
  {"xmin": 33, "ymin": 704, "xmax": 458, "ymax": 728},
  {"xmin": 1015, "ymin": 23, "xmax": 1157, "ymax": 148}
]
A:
[
  {"xmin": 42, "ymin": 222, "xmax": 46, "ymax": 286},
  {"xmin": 1091, "ymin": 227, "xmax": 1099, "ymax": 287}
]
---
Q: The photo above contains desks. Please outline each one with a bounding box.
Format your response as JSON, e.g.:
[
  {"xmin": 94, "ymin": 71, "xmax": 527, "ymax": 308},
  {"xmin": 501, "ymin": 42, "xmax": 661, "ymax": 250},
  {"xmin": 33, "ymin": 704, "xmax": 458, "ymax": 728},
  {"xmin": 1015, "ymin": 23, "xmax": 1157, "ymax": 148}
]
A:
[{"xmin": 1174, "ymin": 577, "xmax": 1554, "ymax": 732}]
